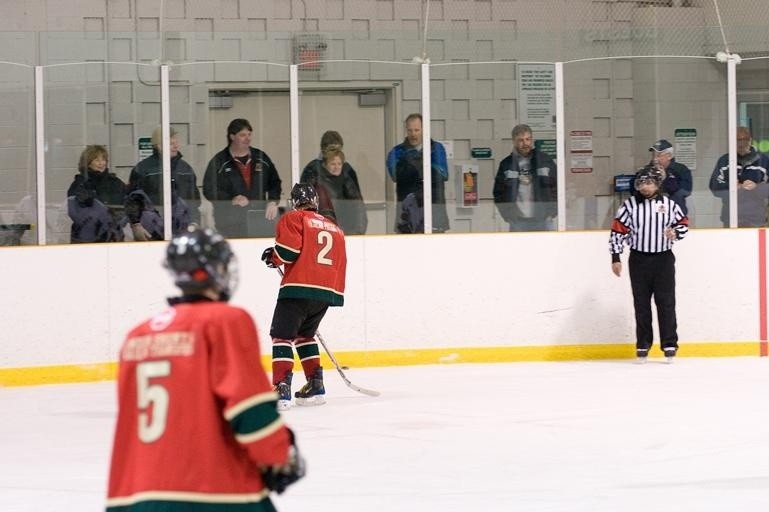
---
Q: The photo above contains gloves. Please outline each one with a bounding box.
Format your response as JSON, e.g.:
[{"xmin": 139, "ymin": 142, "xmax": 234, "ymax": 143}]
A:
[
  {"xmin": 261, "ymin": 248, "xmax": 279, "ymax": 268},
  {"xmin": 263, "ymin": 444, "xmax": 305, "ymax": 494},
  {"xmin": 130, "ymin": 223, "xmax": 152, "ymax": 241}
]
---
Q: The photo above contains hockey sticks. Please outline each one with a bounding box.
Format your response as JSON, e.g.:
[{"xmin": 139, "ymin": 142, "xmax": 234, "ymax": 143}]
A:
[{"xmin": 275, "ymin": 266, "xmax": 380, "ymax": 396}]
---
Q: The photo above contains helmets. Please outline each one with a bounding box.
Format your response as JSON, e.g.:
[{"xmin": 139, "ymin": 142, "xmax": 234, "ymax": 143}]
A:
[
  {"xmin": 166, "ymin": 224, "xmax": 238, "ymax": 301},
  {"xmin": 634, "ymin": 164, "xmax": 663, "ymax": 191},
  {"xmin": 291, "ymin": 181, "xmax": 320, "ymax": 212}
]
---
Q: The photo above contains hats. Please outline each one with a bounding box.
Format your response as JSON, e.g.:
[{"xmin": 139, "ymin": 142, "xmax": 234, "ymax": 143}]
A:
[{"xmin": 649, "ymin": 140, "xmax": 672, "ymax": 152}]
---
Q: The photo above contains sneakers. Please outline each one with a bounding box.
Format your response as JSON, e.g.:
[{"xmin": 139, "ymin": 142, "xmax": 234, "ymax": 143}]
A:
[
  {"xmin": 636, "ymin": 348, "xmax": 648, "ymax": 357},
  {"xmin": 663, "ymin": 348, "xmax": 676, "ymax": 356}
]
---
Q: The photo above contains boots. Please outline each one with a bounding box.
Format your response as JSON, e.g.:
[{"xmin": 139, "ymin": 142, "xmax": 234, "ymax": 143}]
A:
[
  {"xmin": 271, "ymin": 373, "xmax": 292, "ymax": 400},
  {"xmin": 295, "ymin": 366, "xmax": 325, "ymax": 398}
]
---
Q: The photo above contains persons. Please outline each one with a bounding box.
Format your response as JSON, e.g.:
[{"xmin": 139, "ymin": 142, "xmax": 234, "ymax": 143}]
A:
[
  {"xmin": 297, "ymin": 129, "xmax": 362, "ymax": 213},
  {"xmin": 103, "ymin": 221, "xmax": 307, "ymax": 512},
  {"xmin": 708, "ymin": 125, "xmax": 769, "ymax": 228},
  {"xmin": 384, "ymin": 114, "xmax": 450, "ymax": 233},
  {"xmin": 66, "ymin": 142, "xmax": 129, "ymax": 210},
  {"xmin": 303, "ymin": 149, "xmax": 368, "ymax": 235},
  {"xmin": 610, "ymin": 166, "xmax": 692, "ymax": 359},
  {"xmin": 130, "ymin": 123, "xmax": 200, "ymax": 240},
  {"xmin": 12, "ymin": 183, "xmax": 195, "ymax": 245},
  {"xmin": 631, "ymin": 139, "xmax": 693, "ymax": 229},
  {"xmin": 201, "ymin": 116, "xmax": 281, "ymax": 237},
  {"xmin": 259, "ymin": 181, "xmax": 348, "ymax": 399},
  {"xmin": 491, "ymin": 122, "xmax": 558, "ymax": 233}
]
